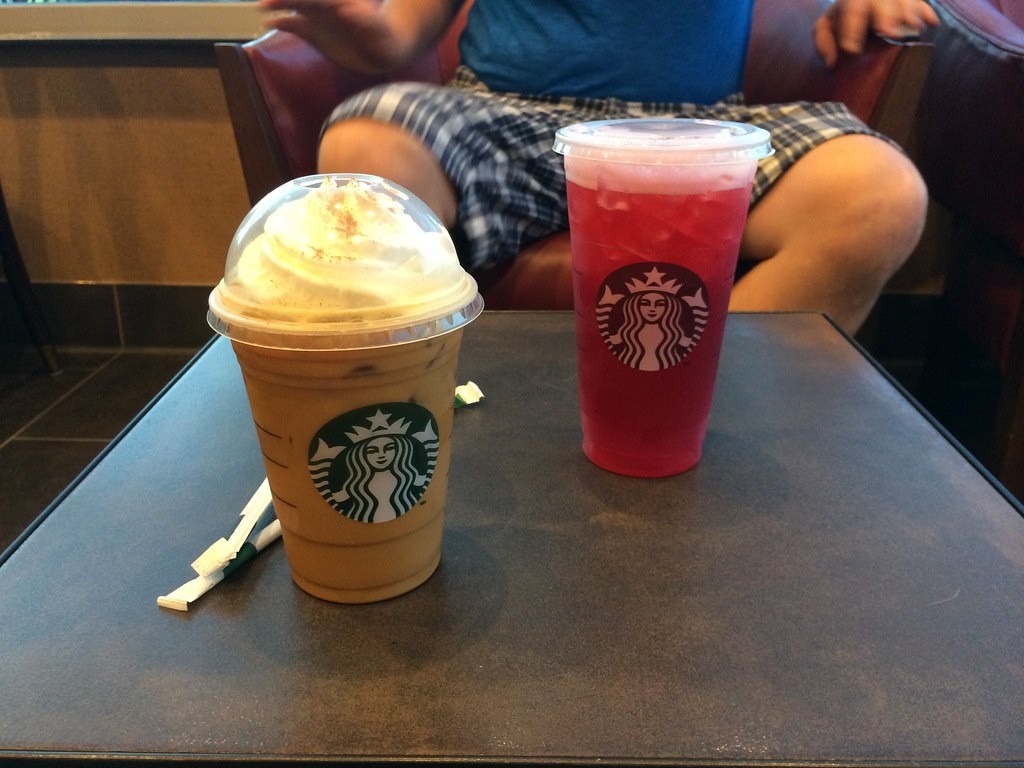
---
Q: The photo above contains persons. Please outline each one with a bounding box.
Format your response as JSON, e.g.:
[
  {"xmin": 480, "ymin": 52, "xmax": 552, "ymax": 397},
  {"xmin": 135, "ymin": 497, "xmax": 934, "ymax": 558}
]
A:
[{"xmin": 254, "ymin": 0, "xmax": 940, "ymax": 339}]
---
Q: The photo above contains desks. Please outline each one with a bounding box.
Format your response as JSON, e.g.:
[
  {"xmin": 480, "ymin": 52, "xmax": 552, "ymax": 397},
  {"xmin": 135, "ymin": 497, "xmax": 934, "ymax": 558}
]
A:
[{"xmin": 0, "ymin": 309, "xmax": 1024, "ymax": 768}]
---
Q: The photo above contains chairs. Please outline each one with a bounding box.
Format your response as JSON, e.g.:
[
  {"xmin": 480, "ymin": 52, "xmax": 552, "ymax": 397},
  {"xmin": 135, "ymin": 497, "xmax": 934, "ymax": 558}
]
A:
[
  {"xmin": 212, "ymin": 0, "xmax": 940, "ymax": 309},
  {"xmin": 912, "ymin": 0, "xmax": 1024, "ymax": 502}
]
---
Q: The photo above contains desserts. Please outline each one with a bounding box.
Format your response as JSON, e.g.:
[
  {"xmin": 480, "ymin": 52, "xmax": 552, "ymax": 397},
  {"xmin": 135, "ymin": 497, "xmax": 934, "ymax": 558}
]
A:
[{"xmin": 226, "ymin": 178, "xmax": 468, "ymax": 603}]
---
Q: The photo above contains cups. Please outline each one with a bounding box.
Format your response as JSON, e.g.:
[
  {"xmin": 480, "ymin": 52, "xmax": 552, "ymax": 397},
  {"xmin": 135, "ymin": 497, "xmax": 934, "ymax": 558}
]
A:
[
  {"xmin": 550, "ymin": 118, "xmax": 775, "ymax": 479},
  {"xmin": 202, "ymin": 174, "xmax": 485, "ymax": 604}
]
